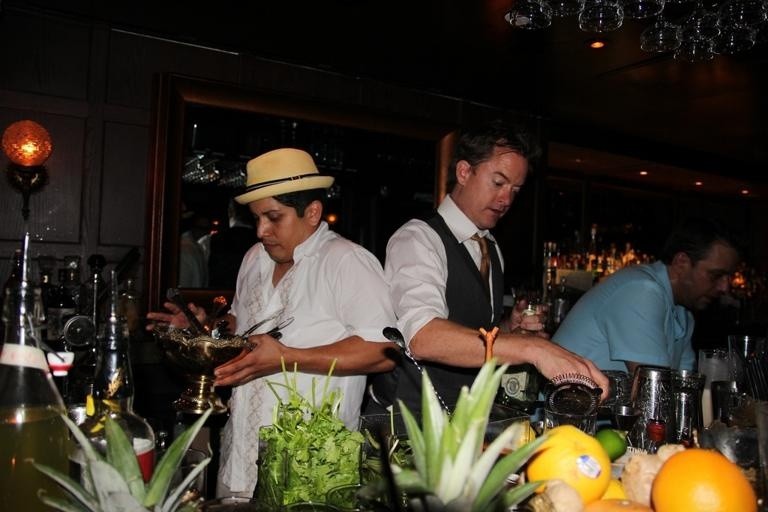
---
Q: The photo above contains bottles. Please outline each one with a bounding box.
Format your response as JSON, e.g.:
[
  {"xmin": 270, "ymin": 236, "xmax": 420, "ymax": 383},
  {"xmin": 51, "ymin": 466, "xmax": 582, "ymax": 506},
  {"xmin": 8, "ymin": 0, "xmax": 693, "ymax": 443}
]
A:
[
  {"xmin": 0, "ymin": 278, "xmax": 70, "ymax": 511},
  {"xmin": 643, "ymin": 422, "xmax": 667, "ymax": 457},
  {"xmin": 544, "ymin": 215, "xmax": 652, "ymax": 274},
  {"xmin": 545, "ymin": 264, "xmax": 557, "ymax": 313},
  {"xmin": 173, "ymin": 397, "xmax": 220, "ymax": 507},
  {"xmin": 70, "ymin": 314, "xmax": 155, "ymax": 492},
  {"xmin": 552, "ymin": 275, "xmax": 568, "ymax": 327},
  {"xmin": 2, "ymin": 250, "xmax": 142, "ymax": 346}
]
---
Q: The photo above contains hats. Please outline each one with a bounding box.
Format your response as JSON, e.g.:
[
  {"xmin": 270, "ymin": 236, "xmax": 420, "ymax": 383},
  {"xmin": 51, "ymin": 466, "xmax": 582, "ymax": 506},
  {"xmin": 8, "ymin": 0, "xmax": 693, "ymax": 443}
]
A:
[{"xmin": 235, "ymin": 149, "xmax": 335, "ymax": 205}]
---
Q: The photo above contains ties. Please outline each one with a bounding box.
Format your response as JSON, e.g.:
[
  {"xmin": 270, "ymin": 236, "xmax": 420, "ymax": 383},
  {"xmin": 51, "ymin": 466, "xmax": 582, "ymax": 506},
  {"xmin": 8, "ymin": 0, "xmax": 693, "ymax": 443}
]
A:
[{"xmin": 473, "ymin": 235, "xmax": 492, "ymax": 285}]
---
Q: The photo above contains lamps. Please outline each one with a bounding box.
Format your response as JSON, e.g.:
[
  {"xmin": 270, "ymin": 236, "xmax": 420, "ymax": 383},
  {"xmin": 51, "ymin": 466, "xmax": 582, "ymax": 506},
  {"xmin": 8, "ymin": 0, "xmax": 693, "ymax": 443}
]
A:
[{"xmin": 2, "ymin": 116, "xmax": 54, "ymax": 223}]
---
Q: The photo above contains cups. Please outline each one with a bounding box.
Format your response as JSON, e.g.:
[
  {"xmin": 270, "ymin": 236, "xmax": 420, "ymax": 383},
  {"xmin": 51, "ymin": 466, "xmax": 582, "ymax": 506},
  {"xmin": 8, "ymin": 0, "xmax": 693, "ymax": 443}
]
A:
[
  {"xmin": 154, "ymin": 447, "xmax": 208, "ymax": 501},
  {"xmin": 600, "ymin": 369, "xmax": 631, "ymax": 409},
  {"xmin": 627, "ymin": 364, "xmax": 674, "ymax": 454},
  {"xmin": 670, "ymin": 368, "xmax": 706, "ymax": 442},
  {"xmin": 709, "ymin": 379, "xmax": 749, "ymax": 425},
  {"xmin": 508, "ymin": 1, "xmax": 767, "ymax": 66},
  {"xmin": 204, "ymin": 495, "xmax": 274, "ymax": 512},
  {"xmin": 518, "ymin": 298, "xmax": 546, "ymax": 338},
  {"xmin": 728, "ymin": 333, "xmax": 766, "ymax": 366},
  {"xmin": 276, "ymin": 500, "xmax": 338, "ymax": 512},
  {"xmin": 604, "ymin": 405, "xmax": 644, "ymax": 430},
  {"xmin": 698, "ymin": 348, "xmax": 731, "ymax": 427}
]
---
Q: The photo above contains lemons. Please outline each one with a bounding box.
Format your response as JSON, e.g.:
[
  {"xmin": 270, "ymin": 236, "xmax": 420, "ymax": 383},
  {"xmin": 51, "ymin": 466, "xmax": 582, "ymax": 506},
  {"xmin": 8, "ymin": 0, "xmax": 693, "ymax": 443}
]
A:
[
  {"xmin": 526, "ymin": 424, "xmax": 611, "ymax": 504},
  {"xmin": 601, "ymin": 478, "xmax": 626, "ymax": 499}
]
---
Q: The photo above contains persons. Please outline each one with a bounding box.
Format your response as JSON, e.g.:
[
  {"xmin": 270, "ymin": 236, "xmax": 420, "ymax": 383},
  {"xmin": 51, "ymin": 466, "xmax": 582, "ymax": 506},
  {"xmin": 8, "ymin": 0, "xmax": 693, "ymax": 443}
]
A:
[
  {"xmin": 141, "ymin": 147, "xmax": 400, "ymax": 510},
  {"xmin": 362, "ymin": 111, "xmax": 611, "ymax": 444},
  {"xmin": 530, "ymin": 209, "xmax": 747, "ymax": 429},
  {"xmin": 177, "ymin": 207, "xmax": 209, "ymax": 289},
  {"xmin": 199, "ymin": 193, "xmax": 261, "ymax": 290}
]
